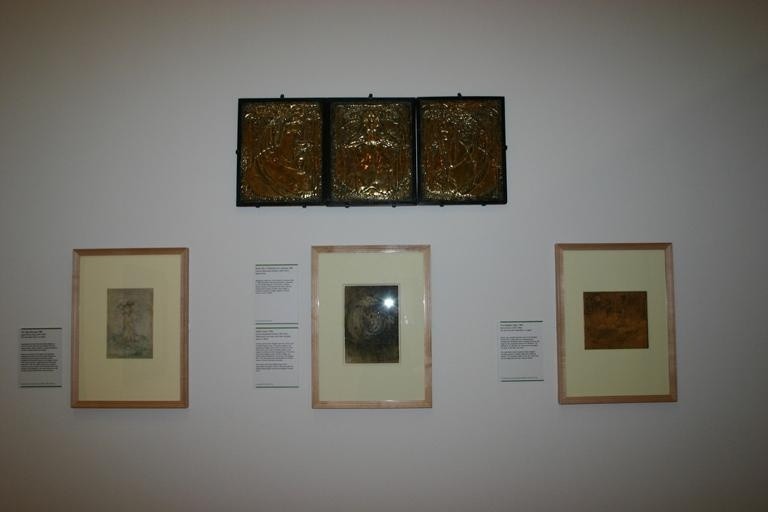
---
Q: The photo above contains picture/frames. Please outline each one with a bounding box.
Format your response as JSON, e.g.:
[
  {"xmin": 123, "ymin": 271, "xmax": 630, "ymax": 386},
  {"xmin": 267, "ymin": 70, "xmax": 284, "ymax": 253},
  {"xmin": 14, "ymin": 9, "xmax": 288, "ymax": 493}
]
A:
[
  {"xmin": 236, "ymin": 93, "xmax": 507, "ymax": 208},
  {"xmin": 311, "ymin": 245, "xmax": 432, "ymax": 409},
  {"xmin": 71, "ymin": 248, "xmax": 188, "ymax": 409},
  {"xmin": 555, "ymin": 243, "xmax": 677, "ymax": 404}
]
